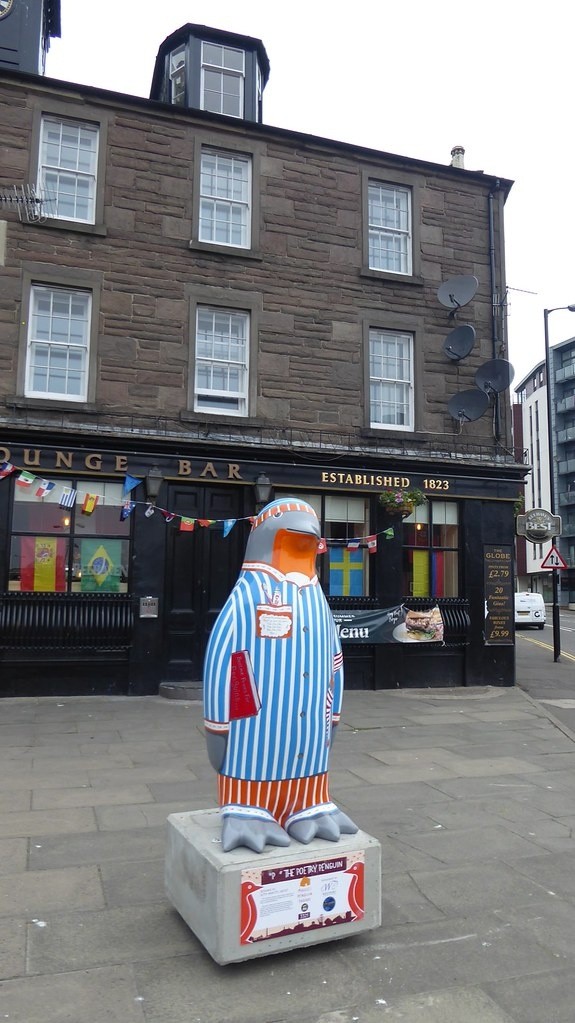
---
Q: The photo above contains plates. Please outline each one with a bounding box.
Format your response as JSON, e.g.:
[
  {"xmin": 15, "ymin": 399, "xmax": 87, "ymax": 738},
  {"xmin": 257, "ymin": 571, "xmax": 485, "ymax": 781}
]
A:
[{"xmin": 393, "ymin": 621, "xmax": 444, "ymax": 643}]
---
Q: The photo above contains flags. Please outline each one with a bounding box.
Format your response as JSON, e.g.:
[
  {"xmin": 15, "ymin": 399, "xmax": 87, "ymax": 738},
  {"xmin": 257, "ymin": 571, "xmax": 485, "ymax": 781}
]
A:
[
  {"xmin": 35, "ymin": 478, "xmax": 55, "ymax": 497},
  {"xmin": 364, "ymin": 535, "xmax": 377, "ymax": 553},
  {"xmin": 15, "ymin": 469, "xmax": 35, "ymax": 487},
  {"xmin": 180, "ymin": 517, "xmax": 195, "ymax": 531},
  {"xmin": 144, "ymin": 503, "xmax": 154, "ymax": 517},
  {"xmin": 329, "ymin": 546, "xmax": 364, "ymax": 597},
  {"xmin": 413, "ymin": 548, "xmax": 446, "ymax": 598},
  {"xmin": 82, "ymin": 493, "xmax": 99, "ymax": 512},
  {"xmin": 346, "ymin": 538, "xmax": 362, "ymax": 552},
  {"xmin": 59, "ymin": 486, "xmax": 77, "ymax": 507},
  {"xmin": 316, "ymin": 537, "xmax": 327, "ymax": 555},
  {"xmin": 0, "ymin": 461, "xmax": 15, "ymax": 480},
  {"xmin": 80, "ymin": 535, "xmax": 122, "ymax": 592},
  {"xmin": 19, "ymin": 535, "xmax": 67, "ymax": 592}
]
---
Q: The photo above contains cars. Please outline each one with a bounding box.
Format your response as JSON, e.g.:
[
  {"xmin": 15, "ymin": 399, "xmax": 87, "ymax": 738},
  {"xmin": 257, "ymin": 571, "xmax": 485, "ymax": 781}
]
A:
[{"xmin": 515, "ymin": 592, "xmax": 546, "ymax": 629}]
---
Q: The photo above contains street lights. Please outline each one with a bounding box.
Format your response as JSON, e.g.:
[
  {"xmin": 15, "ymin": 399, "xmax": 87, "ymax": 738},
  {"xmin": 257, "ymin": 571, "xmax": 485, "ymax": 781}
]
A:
[{"xmin": 544, "ymin": 304, "xmax": 575, "ymax": 663}]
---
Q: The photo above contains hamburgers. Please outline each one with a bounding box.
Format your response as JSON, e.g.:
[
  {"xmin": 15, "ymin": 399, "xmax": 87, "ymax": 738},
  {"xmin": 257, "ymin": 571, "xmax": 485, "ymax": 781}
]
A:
[{"xmin": 405, "ymin": 611, "xmax": 439, "ymax": 640}]
